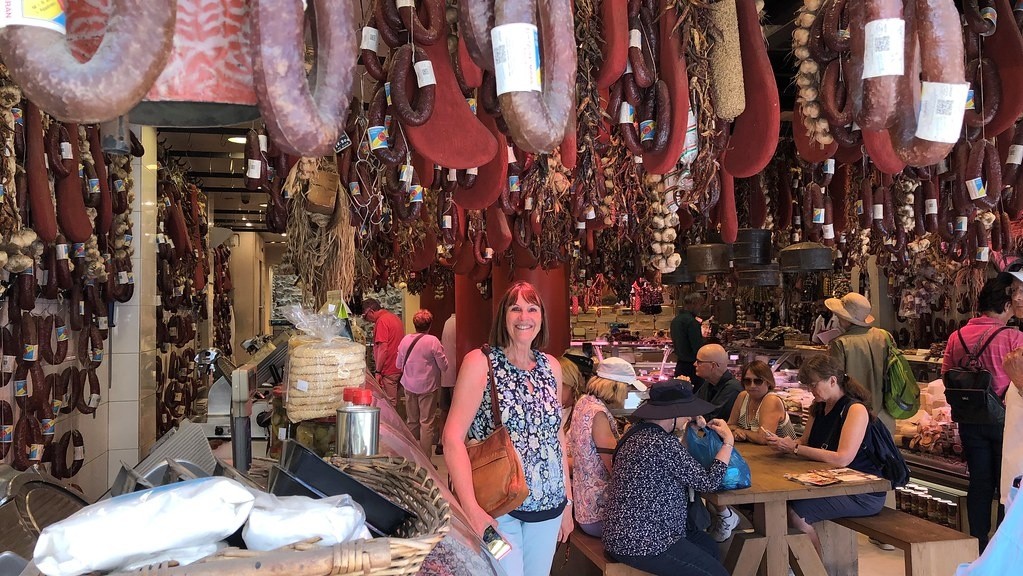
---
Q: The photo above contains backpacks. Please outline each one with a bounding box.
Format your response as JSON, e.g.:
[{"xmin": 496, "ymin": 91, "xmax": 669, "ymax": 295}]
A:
[
  {"xmin": 879, "ymin": 329, "xmax": 920, "ymax": 419},
  {"xmin": 840, "ymin": 400, "xmax": 911, "ymax": 487},
  {"xmin": 944, "ymin": 326, "xmax": 1014, "ymax": 425}
]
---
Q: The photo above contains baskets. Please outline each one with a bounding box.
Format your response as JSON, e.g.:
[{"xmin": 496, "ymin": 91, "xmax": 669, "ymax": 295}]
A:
[{"xmin": 110, "ymin": 452, "xmax": 451, "ymax": 576}]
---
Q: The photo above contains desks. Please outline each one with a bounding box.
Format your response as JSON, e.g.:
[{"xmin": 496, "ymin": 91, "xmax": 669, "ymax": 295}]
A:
[{"xmin": 603, "ymin": 406, "xmax": 891, "ymax": 576}]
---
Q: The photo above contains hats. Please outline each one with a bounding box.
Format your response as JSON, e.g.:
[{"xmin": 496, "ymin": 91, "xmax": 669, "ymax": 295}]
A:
[
  {"xmin": 824, "ymin": 292, "xmax": 875, "ymax": 327},
  {"xmin": 597, "ymin": 357, "xmax": 647, "ymax": 392},
  {"xmin": 632, "ymin": 379, "xmax": 715, "ymax": 420},
  {"xmin": 1008, "ymin": 267, "xmax": 1023, "ymax": 282}
]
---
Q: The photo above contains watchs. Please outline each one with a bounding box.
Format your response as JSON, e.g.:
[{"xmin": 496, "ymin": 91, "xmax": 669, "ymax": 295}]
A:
[
  {"xmin": 793, "ymin": 444, "xmax": 799, "ymax": 457},
  {"xmin": 566, "ymin": 498, "xmax": 574, "ymax": 506}
]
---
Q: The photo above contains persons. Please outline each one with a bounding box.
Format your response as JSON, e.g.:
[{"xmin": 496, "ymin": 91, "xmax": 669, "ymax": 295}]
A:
[
  {"xmin": 553, "ymin": 356, "xmax": 583, "ymax": 496},
  {"xmin": 571, "ymin": 357, "xmax": 649, "ymax": 535},
  {"xmin": 762, "ymin": 353, "xmax": 888, "ymax": 562},
  {"xmin": 706, "ymin": 360, "xmax": 799, "ymax": 543},
  {"xmin": 602, "ymin": 380, "xmax": 730, "ymax": 576},
  {"xmin": 435, "ymin": 313, "xmax": 456, "ymax": 455},
  {"xmin": 440, "ymin": 282, "xmax": 575, "ymax": 576},
  {"xmin": 670, "ymin": 293, "xmax": 706, "ymax": 395},
  {"xmin": 361, "ymin": 298, "xmax": 404, "ymax": 409},
  {"xmin": 939, "ymin": 265, "xmax": 1023, "ymax": 558},
  {"xmin": 824, "ymin": 292, "xmax": 896, "ymax": 512},
  {"xmin": 396, "ymin": 310, "xmax": 449, "ymax": 471},
  {"xmin": 811, "ymin": 294, "xmax": 841, "ymax": 345},
  {"xmin": 692, "ymin": 344, "xmax": 748, "ymax": 442}
]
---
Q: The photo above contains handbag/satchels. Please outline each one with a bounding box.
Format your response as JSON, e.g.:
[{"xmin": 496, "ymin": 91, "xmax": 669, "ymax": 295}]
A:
[
  {"xmin": 686, "ymin": 420, "xmax": 751, "ymax": 492},
  {"xmin": 447, "ymin": 343, "xmax": 528, "ymax": 519}
]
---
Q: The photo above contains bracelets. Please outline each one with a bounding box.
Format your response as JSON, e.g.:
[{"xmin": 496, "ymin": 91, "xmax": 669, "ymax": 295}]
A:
[
  {"xmin": 374, "ymin": 370, "xmax": 381, "ymax": 373},
  {"xmin": 723, "ymin": 442, "xmax": 734, "ymax": 447}
]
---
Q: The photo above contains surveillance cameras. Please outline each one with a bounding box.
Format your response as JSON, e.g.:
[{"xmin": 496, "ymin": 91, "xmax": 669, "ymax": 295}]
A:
[{"xmin": 241, "ymin": 196, "xmax": 250, "ymax": 204}]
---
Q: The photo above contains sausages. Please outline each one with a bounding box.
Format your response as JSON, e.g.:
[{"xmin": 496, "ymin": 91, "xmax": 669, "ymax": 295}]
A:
[{"xmin": 0, "ymin": 0, "xmax": 1023, "ymax": 479}]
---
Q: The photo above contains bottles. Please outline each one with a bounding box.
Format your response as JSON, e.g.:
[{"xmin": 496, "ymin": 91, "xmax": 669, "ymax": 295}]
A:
[
  {"xmin": 342, "ymin": 387, "xmax": 372, "ymax": 407},
  {"xmin": 271, "ymin": 386, "xmax": 337, "ymax": 460}
]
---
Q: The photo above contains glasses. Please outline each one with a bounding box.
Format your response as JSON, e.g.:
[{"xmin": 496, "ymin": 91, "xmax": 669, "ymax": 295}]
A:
[
  {"xmin": 1006, "ymin": 286, "xmax": 1023, "ymax": 296},
  {"xmin": 741, "ymin": 377, "xmax": 763, "ymax": 386},
  {"xmin": 695, "ymin": 359, "xmax": 719, "ymax": 367},
  {"xmin": 799, "ymin": 378, "xmax": 824, "ymax": 390}
]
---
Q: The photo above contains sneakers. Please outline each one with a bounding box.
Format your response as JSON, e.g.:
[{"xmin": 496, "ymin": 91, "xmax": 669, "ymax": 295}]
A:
[{"xmin": 706, "ymin": 502, "xmax": 741, "ymax": 543}]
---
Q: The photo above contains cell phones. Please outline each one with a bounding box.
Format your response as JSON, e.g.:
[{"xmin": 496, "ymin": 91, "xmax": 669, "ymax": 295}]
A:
[
  {"xmin": 761, "ymin": 427, "xmax": 772, "ymax": 438},
  {"xmin": 483, "ymin": 525, "xmax": 511, "ymax": 560}
]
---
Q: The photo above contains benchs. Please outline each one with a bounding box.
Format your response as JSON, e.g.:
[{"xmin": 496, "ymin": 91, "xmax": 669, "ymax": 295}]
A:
[
  {"xmin": 827, "ymin": 506, "xmax": 978, "ymax": 576},
  {"xmin": 570, "ymin": 525, "xmax": 656, "ymax": 576}
]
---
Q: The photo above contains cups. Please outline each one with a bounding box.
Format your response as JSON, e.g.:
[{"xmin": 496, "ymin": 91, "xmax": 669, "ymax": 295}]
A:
[{"xmin": 336, "ymin": 406, "xmax": 380, "ymax": 457}]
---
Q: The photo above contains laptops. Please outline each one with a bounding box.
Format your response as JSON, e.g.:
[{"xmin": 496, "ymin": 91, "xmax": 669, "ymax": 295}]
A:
[{"xmin": 611, "ymin": 390, "xmax": 647, "ymax": 418}]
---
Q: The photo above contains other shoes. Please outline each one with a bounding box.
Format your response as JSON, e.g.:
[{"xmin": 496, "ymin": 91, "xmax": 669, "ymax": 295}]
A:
[
  {"xmin": 868, "ymin": 537, "xmax": 895, "ymax": 550},
  {"xmin": 435, "ymin": 444, "xmax": 444, "ymax": 455}
]
